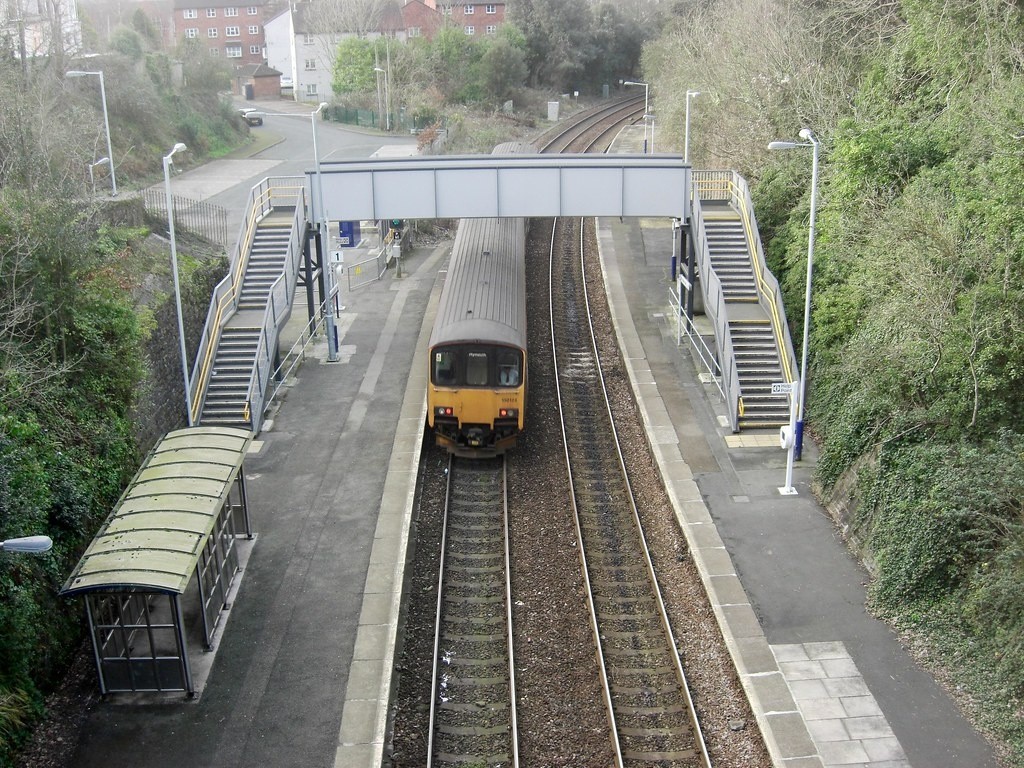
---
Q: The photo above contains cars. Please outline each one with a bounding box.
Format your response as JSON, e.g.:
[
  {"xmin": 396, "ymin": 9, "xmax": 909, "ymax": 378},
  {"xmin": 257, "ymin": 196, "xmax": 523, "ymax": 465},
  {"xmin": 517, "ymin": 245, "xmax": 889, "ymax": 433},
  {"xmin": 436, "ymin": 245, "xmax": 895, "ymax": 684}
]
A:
[{"xmin": 239, "ymin": 108, "xmax": 263, "ymax": 127}]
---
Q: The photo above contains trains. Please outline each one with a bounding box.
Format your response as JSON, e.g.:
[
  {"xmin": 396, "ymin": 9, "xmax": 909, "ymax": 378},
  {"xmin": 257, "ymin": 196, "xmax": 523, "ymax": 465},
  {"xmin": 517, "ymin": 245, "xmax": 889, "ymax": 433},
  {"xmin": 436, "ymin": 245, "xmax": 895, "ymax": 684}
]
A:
[{"xmin": 425, "ymin": 142, "xmax": 540, "ymax": 461}]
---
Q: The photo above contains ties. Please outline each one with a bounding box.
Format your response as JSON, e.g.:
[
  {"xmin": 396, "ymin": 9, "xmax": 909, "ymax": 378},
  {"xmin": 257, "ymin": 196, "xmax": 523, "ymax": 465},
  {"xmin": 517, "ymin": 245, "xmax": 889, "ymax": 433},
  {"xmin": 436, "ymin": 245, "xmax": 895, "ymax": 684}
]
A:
[{"xmin": 506, "ymin": 373, "xmax": 509, "ymax": 383}]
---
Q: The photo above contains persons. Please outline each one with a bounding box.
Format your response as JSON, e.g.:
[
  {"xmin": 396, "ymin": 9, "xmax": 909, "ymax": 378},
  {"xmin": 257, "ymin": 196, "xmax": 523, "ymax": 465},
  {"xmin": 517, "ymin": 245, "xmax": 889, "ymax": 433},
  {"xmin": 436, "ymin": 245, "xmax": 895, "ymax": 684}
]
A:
[{"xmin": 500, "ymin": 367, "xmax": 518, "ymax": 385}]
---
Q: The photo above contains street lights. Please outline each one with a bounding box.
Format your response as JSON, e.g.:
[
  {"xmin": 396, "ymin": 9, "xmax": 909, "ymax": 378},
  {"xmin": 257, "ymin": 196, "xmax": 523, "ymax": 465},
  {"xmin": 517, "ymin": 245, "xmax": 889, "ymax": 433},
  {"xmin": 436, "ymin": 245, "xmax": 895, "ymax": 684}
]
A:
[
  {"xmin": 767, "ymin": 128, "xmax": 817, "ymax": 461},
  {"xmin": 162, "ymin": 143, "xmax": 194, "ymax": 428},
  {"xmin": 88, "ymin": 157, "xmax": 110, "ymax": 198},
  {"xmin": 623, "ymin": 81, "xmax": 648, "ymax": 153},
  {"xmin": 684, "ymin": 89, "xmax": 702, "ymax": 163},
  {"xmin": 311, "ymin": 101, "xmax": 341, "ymax": 361},
  {"xmin": 642, "ymin": 115, "xmax": 655, "ymax": 154},
  {"xmin": 66, "ymin": 69, "xmax": 118, "ymax": 197}
]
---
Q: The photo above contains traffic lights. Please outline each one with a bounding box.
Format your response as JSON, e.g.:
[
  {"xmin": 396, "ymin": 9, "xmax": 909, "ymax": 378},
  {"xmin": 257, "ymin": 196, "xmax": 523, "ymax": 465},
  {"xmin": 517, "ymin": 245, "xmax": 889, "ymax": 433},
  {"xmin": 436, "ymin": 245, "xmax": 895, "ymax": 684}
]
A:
[{"xmin": 389, "ymin": 219, "xmax": 404, "ymax": 229}]
